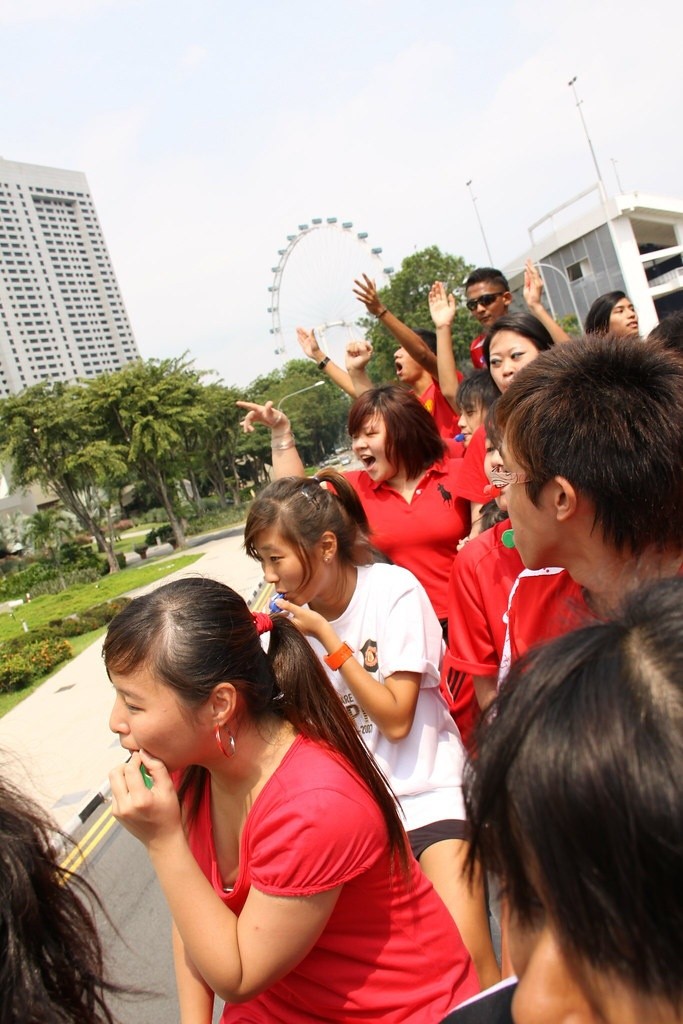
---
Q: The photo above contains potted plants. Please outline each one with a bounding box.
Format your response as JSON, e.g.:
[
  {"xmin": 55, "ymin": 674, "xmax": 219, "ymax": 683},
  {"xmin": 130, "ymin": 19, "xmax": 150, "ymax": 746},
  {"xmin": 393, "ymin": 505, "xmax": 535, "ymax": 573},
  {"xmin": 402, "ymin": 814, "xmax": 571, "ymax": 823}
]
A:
[
  {"xmin": 135, "ymin": 546, "xmax": 148, "ymax": 559},
  {"xmin": 167, "ymin": 536, "xmax": 177, "ymax": 550}
]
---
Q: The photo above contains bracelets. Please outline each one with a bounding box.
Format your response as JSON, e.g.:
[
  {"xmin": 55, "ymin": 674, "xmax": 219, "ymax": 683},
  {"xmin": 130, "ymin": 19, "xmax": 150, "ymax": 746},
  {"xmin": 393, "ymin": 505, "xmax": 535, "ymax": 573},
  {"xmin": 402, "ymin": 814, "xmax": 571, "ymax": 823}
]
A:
[
  {"xmin": 324, "ymin": 640, "xmax": 356, "ymax": 672},
  {"xmin": 267, "ymin": 411, "xmax": 295, "ymax": 451},
  {"xmin": 318, "ymin": 356, "xmax": 331, "ymax": 369},
  {"xmin": 376, "ymin": 307, "xmax": 387, "ymax": 318}
]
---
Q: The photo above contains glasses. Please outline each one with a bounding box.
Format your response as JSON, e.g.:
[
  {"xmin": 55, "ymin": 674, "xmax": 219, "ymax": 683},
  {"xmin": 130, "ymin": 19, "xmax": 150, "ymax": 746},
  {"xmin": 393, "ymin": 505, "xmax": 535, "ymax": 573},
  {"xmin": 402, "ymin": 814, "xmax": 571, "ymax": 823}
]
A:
[
  {"xmin": 491, "ymin": 465, "xmax": 532, "ymax": 488},
  {"xmin": 467, "ymin": 293, "xmax": 503, "ymax": 311}
]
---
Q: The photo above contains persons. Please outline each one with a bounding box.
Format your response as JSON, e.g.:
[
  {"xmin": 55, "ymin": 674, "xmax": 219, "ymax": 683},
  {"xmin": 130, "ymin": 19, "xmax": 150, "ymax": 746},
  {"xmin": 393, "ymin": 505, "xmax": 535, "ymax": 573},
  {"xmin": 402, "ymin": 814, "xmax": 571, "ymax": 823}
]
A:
[
  {"xmin": 0, "ymin": 770, "xmax": 169, "ymax": 1024},
  {"xmin": 239, "ymin": 261, "xmax": 683, "ymax": 1024},
  {"xmin": 100, "ymin": 578, "xmax": 481, "ymax": 1024}
]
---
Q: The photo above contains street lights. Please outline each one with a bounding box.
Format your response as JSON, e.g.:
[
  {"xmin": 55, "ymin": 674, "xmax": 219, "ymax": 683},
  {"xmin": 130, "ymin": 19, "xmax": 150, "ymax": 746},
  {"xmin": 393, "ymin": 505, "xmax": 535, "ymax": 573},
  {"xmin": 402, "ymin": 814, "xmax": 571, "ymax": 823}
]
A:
[
  {"xmin": 467, "ymin": 181, "xmax": 495, "ymax": 267},
  {"xmin": 569, "ymin": 77, "xmax": 602, "ymax": 181}
]
